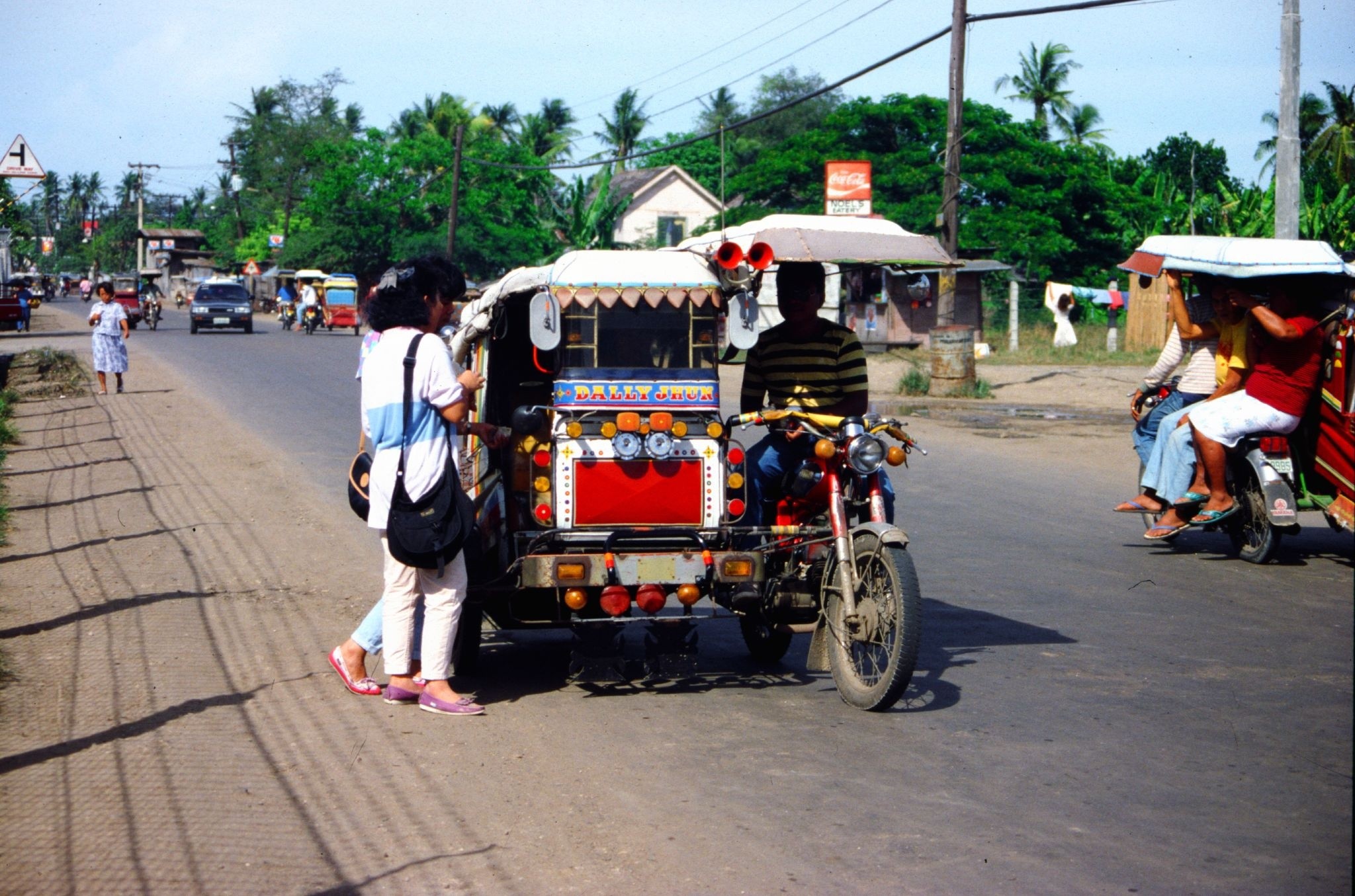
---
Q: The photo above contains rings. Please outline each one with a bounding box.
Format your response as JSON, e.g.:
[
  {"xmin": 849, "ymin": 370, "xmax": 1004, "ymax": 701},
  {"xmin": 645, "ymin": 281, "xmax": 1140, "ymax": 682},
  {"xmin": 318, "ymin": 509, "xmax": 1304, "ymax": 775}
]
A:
[{"xmin": 1132, "ymin": 407, "xmax": 1135, "ymax": 409}]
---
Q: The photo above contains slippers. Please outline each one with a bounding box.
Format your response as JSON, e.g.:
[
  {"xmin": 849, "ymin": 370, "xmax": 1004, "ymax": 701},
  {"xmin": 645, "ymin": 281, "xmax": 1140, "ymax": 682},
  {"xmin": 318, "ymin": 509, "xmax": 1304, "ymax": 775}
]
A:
[
  {"xmin": 1112, "ymin": 499, "xmax": 1162, "ymax": 514},
  {"xmin": 1173, "ymin": 492, "xmax": 1211, "ymax": 505},
  {"xmin": 1190, "ymin": 503, "xmax": 1240, "ymax": 523},
  {"xmin": 1143, "ymin": 524, "xmax": 1195, "ymax": 539}
]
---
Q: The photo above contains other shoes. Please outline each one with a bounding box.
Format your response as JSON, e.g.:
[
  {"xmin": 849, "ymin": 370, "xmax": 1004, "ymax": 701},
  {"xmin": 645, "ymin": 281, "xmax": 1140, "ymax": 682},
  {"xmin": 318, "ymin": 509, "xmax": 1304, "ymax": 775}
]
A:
[
  {"xmin": 412, "ymin": 677, "xmax": 428, "ymax": 688},
  {"xmin": 418, "ymin": 690, "xmax": 485, "ymax": 716},
  {"xmin": 328, "ymin": 645, "xmax": 381, "ymax": 695},
  {"xmin": 382, "ymin": 685, "xmax": 419, "ymax": 704},
  {"xmin": 117, "ymin": 379, "xmax": 123, "ymax": 393},
  {"xmin": 99, "ymin": 391, "xmax": 107, "ymax": 394}
]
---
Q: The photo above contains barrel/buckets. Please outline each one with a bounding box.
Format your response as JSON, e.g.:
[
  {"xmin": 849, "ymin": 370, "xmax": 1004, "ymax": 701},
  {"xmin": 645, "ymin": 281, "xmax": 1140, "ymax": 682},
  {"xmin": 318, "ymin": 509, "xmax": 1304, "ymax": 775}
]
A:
[{"xmin": 928, "ymin": 325, "xmax": 975, "ymax": 394}]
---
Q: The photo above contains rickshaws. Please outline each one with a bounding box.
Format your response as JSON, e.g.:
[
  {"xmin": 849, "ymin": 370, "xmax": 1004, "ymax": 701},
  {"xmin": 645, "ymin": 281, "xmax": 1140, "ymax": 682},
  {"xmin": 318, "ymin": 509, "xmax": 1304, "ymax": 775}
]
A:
[
  {"xmin": 270, "ymin": 269, "xmax": 361, "ymax": 337},
  {"xmin": 111, "ymin": 269, "xmax": 166, "ymax": 330},
  {"xmin": 454, "ymin": 211, "xmax": 963, "ymax": 716},
  {"xmin": 1118, "ymin": 236, "xmax": 1355, "ymax": 567}
]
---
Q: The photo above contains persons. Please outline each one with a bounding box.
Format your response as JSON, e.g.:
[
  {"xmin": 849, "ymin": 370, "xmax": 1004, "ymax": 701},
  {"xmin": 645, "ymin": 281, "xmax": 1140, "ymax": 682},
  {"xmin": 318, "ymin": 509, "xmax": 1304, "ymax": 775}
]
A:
[
  {"xmin": 88, "ymin": 281, "xmax": 129, "ymax": 394},
  {"xmin": 42, "ymin": 276, "xmax": 51, "ymax": 298},
  {"xmin": 59, "ymin": 279, "xmax": 70, "ymax": 295},
  {"xmin": 273, "ymin": 278, "xmax": 322, "ymax": 331},
  {"xmin": 732, "ymin": 260, "xmax": 895, "ymax": 606},
  {"xmin": 140, "ymin": 275, "xmax": 166, "ymax": 320},
  {"xmin": 80, "ymin": 276, "xmax": 93, "ymax": 300},
  {"xmin": 327, "ymin": 257, "xmax": 485, "ymax": 715},
  {"xmin": 1113, "ymin": 269, "xmax": 1339, "ymax": 539},
  {"xmin": 1047, "ymin": 281, "xmax": 1077, "ymax": 347},
  {"xmin": 13, "ymin": 283, "xmax": 33, "ymax": 331}
]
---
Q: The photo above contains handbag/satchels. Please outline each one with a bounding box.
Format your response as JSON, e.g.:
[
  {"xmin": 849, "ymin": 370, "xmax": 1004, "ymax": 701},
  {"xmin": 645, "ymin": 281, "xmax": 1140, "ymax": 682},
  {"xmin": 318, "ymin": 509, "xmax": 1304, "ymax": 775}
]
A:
[
  {"xmin": 387, "ymin": 458, "xmax": 475, "ymax": 577},
  {"xmin": 348, "ymin": 453, "xmax": 375, "ymax": 521}
]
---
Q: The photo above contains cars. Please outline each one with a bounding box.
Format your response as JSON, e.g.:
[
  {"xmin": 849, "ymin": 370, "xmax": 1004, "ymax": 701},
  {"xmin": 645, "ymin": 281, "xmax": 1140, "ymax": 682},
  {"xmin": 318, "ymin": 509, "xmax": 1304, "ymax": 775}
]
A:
[{"xmin": 189, "ymin": 282, "xmax": 255, "ymax": 334}]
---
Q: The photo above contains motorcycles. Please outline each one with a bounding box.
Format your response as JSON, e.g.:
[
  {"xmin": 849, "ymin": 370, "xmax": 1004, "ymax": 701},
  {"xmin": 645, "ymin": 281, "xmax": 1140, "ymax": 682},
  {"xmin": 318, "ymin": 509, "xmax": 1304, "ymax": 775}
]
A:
[{"xmin": 79, "ymin": 292, "xmax": 93, "ymax": 301}]
